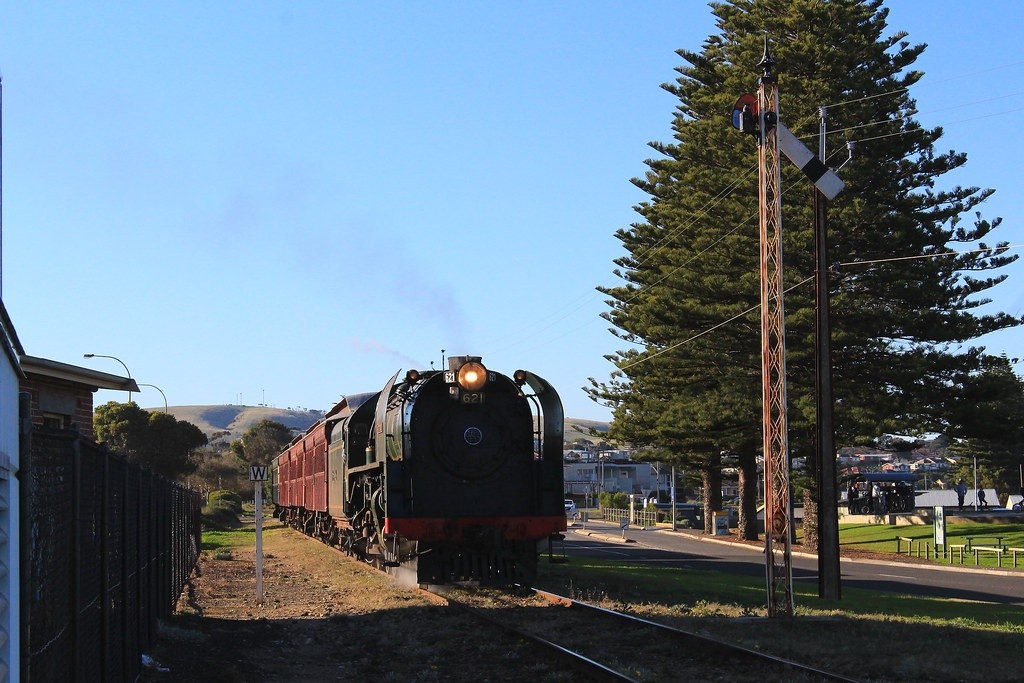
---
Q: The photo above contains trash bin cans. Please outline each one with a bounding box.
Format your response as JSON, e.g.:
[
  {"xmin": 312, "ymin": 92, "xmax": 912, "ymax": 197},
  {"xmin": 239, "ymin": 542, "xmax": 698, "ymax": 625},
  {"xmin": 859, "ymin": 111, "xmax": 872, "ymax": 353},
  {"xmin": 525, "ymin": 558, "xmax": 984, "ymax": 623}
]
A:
[
  {"xmin": 578, "ymin": 511, "xmax": 588, "ymax": 522},
  {"xmin": 712, "ymin": 510, "xmax": 730, "ymax": 536}
]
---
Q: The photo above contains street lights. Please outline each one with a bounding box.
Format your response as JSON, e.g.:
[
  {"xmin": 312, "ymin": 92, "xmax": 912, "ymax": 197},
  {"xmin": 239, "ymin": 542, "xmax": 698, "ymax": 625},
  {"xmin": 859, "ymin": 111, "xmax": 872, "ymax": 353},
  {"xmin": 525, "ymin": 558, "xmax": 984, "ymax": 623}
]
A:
[{"xmin": 85, "ymin": 351, "xmax": 133, "ymax": 403}]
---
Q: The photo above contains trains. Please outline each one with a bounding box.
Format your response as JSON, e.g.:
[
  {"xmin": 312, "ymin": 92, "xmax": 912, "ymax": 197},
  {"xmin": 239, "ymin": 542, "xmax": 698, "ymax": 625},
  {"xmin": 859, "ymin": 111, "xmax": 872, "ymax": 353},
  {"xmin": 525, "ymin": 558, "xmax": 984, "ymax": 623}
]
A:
[{"xmin": 271, "ymin": 351, "xmax": 569, "ymax": 593}]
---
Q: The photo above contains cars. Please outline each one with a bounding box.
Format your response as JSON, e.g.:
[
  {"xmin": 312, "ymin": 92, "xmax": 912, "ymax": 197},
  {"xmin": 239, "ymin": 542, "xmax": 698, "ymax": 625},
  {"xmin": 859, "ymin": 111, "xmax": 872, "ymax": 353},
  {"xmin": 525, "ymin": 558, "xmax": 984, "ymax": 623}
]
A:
[{"xmin": 564, "ymin": 499, "xmax": 577, "ymax": 515}]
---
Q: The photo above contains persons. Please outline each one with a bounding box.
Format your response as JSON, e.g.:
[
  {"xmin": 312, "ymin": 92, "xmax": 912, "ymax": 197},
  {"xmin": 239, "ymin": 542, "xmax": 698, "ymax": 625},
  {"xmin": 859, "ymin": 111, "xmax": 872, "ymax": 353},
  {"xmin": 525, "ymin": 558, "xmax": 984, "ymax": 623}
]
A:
[
  {"xmin": 978, "ymin": 488, "xmax": 989, "ymax": 509},
  {"xmin": 954, "ymin": 480, "xmax": 967, "ymax": 510},
  {"xmin": 847, "ymin": 481, "xmax": 913, "ymax": 515}
]
